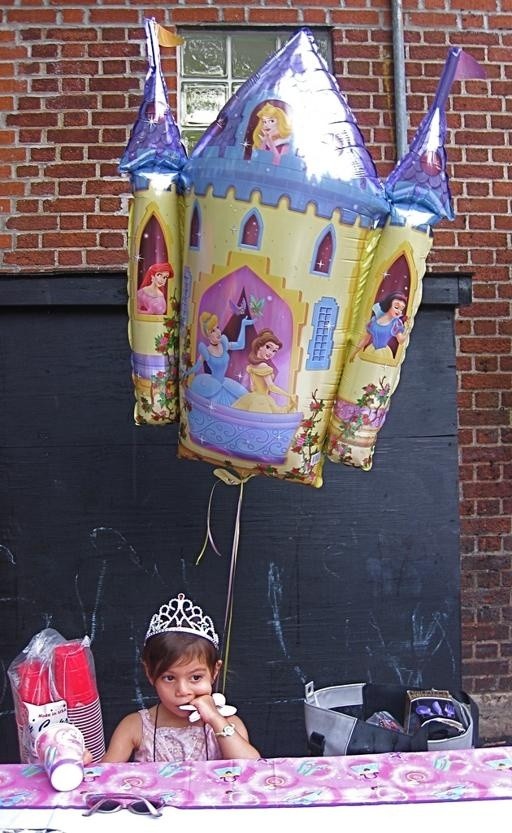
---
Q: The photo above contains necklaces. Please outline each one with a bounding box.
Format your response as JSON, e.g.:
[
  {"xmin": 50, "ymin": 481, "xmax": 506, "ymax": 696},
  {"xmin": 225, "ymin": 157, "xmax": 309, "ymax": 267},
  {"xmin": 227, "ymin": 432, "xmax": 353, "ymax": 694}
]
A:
[{"xmin": 153, "ymin": 698, "xmax": 209, "ymax": 762}]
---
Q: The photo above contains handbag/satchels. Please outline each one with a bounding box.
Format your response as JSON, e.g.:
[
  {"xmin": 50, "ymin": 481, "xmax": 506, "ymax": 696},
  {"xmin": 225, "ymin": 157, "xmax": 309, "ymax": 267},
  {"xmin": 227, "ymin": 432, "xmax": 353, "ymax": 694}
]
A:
[{"xmin": 300, "ymin": 678, "xmax": 480, "ymax": 756}]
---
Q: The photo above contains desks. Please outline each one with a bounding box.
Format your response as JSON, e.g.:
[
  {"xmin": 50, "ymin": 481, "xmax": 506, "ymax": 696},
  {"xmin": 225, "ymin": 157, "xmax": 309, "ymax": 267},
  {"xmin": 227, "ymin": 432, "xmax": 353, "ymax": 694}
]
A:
[{"xmin": 1, "ymin": 746, "xmax": 512, "ymax": 833}]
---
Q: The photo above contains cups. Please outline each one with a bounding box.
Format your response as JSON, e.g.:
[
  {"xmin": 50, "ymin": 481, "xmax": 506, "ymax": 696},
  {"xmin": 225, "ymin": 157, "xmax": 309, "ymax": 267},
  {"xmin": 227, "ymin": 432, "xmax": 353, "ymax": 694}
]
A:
[
  {"xmin": 33, "ymin": 722, "xmax": 85, "ymax": 793},
  {"xmin": 14, "ymin": 642, "xmax": 106, "ymax": 766}
]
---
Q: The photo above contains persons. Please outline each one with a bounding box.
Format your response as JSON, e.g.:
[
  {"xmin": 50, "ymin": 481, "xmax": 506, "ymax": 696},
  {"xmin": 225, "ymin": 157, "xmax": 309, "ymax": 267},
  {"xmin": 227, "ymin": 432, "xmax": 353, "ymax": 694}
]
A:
[{"xmin": 99, "ymin": 592, "xmax": 262, "ymax": 765}]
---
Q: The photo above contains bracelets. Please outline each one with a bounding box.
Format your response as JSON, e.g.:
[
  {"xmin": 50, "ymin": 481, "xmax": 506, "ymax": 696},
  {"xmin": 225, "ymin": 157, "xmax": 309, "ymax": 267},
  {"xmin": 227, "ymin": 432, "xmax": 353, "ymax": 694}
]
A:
[{"xmin": 214, "ymin": 722, "xmax": 237, "ymax": 737}]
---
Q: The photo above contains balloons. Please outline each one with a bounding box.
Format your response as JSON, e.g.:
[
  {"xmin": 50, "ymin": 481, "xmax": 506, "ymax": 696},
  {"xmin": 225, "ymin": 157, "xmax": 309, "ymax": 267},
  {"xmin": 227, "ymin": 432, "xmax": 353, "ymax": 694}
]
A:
[{"xmin": 119, "ymin": 12, "xmax": 489, "ymax": 487}]
